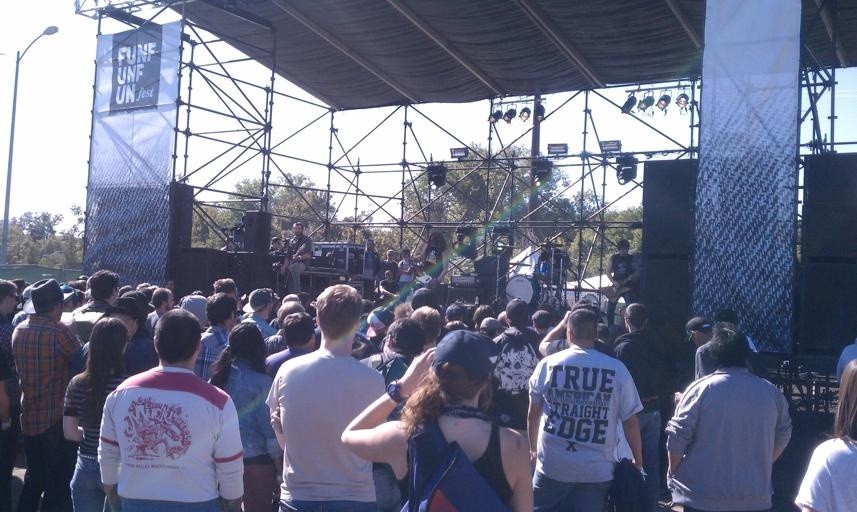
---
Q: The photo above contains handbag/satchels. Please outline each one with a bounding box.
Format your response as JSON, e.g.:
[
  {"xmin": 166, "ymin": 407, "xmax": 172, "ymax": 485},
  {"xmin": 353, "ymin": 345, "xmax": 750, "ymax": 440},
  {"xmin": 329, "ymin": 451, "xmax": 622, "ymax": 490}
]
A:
[{"xmin": 400, "ymin": 441, "xmax": 511, "ymax": 512}]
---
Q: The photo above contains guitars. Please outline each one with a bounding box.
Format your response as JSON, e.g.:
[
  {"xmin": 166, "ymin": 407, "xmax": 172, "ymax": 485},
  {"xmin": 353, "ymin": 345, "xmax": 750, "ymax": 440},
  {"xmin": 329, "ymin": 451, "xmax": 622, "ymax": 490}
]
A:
[
  {"xmin": 281, "ymin": 243, "xmax": 306, "ymax": 274},
  {"xmin": 597, "ymin": 272, "xmax": 640, "ymax": 304},
  {"xmin": 409, "ymin": 260, "xmax": 432, "ymax": 284}
]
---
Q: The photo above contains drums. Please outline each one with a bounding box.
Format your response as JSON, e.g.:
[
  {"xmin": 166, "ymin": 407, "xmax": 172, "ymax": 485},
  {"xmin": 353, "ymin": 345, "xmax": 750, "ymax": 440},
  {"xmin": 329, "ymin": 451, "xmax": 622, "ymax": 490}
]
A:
[
  {"xmin": 535, "ymin": 262, "xmax": 551, "ymax": 276},
  {"xmin": 504, "ymin": 274, "xmax": 545, "ymax": 307}
]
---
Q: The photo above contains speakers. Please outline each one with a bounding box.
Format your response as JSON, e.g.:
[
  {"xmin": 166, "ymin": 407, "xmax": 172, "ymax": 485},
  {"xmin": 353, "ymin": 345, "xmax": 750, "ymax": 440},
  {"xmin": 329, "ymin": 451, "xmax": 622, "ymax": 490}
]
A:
[
  {"xmin": 174, "ymin": 248, "xmax": 228, "ymax": 304},
  {"xmin": 228, "ymin": 252, "xmax": 273, "ymax": 302},
  {"xmin": 242, "ymin": 210, "xmax": 271, "ymax": 252},
  {"xmin": 642, "ymin": 158, "xmax": 698, "ymax": 258},
  {"xmin": 641, "ymin": 257, "xmax": 693, "ymax": 339},
  {"xmin": 801, "ymin": 152, "xmax": 857, "ymax": 260},
  {"xmin": 795, "ymin": 259, "xmax": 856, "ymax": 374}
]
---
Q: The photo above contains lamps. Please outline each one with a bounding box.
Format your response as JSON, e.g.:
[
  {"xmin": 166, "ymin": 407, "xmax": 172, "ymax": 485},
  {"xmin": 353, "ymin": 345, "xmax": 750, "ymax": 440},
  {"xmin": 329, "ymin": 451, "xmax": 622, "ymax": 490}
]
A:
[
  {"xmin": 532, "ymin": 161, "xmax": 552, "ymax": 179},
  {"xmin": 488, "ymin": 106, "xmax": 503, "ymax": 123},
  {"xmin": 615, "ymin": 155, "xmax": 637, "ymax": 184},
  {"xmin": 638, "ymin": 96, "xmax": 654, "ymax": 111},
  {"xmin": 676, "ymin": 93, "xmax": 689, "ymax": 112},
  {"xmin": 518, "ymin": 106, "xmax": 531, "ymax": 123},
  {"xmin": 503, "ymin": 106, "xmax": 518, "ymax": 123},
  {"xmin": 451, "ymin": 147, "xmax": 468, "ymax": 160},
  {"xmin": 547, "ymin": 144, "xmax": 569, "ymax": 156},
  {"xmin": 621, "ymin": 96, "xmax": 637, "ymax": 113},
  {"xmin": 656, "ymin": 95, "xmax": 671, "ymax": 111},
  {"xmin": 427, "ymin": 165, "xmax": 447, "ymax": 187},
  {"xmin": 598, "ymin": 141, "xmax": 621, "ymax": 154},
  {"xmin": 534, "ymin": 104, "xmax": 545, "ymax": 122}
]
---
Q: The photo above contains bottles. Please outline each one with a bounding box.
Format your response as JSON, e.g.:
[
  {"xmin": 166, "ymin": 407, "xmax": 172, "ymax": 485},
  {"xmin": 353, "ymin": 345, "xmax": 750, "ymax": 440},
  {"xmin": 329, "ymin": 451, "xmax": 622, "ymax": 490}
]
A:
[{"xmin": 475, "ymin": 295, "xmax": 479, "ymax": 305}]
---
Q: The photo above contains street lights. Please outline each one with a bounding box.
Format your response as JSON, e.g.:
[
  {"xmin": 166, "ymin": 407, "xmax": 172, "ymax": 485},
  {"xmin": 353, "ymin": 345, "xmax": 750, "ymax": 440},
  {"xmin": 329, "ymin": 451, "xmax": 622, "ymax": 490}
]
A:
[{"xmin": 1, "ymin": 24, "xmax": 60, "ymax": 267}]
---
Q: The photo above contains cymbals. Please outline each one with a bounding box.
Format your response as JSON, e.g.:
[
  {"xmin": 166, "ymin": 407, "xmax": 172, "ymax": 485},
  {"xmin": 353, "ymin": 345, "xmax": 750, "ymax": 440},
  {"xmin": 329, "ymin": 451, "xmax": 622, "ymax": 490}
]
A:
[
  {"xmin": 510, "ymin": 263, "xmax": 532, "ymax": 266},
  {"xmin": 554, "ymin": 252, "xmax": 565, "ymax": 254},
  {"xmin": 540, "ymin": 243, "xmax": 563, "ymax": 247}
]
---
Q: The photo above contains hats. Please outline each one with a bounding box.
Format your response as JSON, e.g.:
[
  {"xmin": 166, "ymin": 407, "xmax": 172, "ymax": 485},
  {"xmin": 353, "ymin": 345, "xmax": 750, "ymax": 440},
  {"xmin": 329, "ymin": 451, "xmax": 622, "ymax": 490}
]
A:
[
  {"xmin": 432, "ymin": 330, "xmax": 499, "ymax": 385},
  {"xmin": 16, "ymin": 278, "xmax": 76, "ymax": 315},
  {"xmin": 180, "ymin": 295, "xmax": 209, "ymax": 328},
  {"xmin": 366, "ymin": 306, "xmax": 395, "ymax": 338},
  {"xmin": 686, "ymin": 317, "xmax": 712, "ymax": 343},
  {"xmin": 242, "ymin": 288, "xmax": 271, "ymax": 312},
  {"xmin": 480, "ymin": 317, "xmax": 508, "ymax": 331}
]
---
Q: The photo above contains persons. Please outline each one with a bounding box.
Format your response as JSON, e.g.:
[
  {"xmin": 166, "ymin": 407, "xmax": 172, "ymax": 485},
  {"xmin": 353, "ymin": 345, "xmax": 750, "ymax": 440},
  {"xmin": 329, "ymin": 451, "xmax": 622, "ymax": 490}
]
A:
[
  {"xmin": 2, "ymin": 221, "xmax": 794, "ymax": 511},
  {"xmin": 836, "ymin": 339, "xmax": 857, "ymax": 382},
  {"xmin": 794, "ymin": 358, "xmax": 857, "ymax": 511}
]
